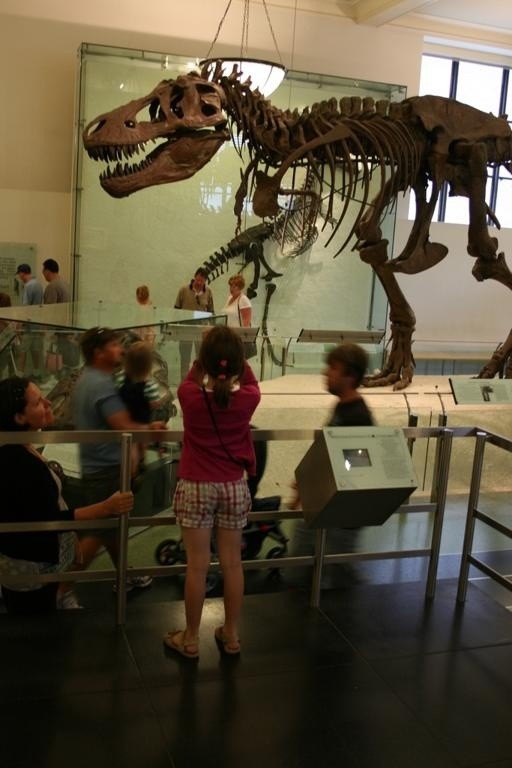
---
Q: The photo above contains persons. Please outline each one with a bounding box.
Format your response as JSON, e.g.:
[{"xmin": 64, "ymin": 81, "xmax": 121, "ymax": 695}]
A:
[
  {"xmin": 41, "ymin": 257, "xmax": 79, "ymax": 385},
  {"xmin": 0, "ymin": 292, "xmax": 14, "ymax": 381},
  {"xmin": 221, "ymin": 273, "xmax": 252, "ymax": 328},
  {"xmin": 62, "ymin": 325, "xmax": 157, "ymax": 588},
  {"xmin": 1, "ymin": 373, "xmax": 134, "ymax": 613},
  {"xmin": 160, "ymin": 323, "xmax": 262, "ymax": 660},
  {"xmin": 114, "ymin": 344, "xmax": 162, "ymax": 424},
  {"xmin": 133, "ymin": 286, "xmax": 156, "ymax": 345},
  {"xmin": 175, "ymin": 268, "xmax": 216, "ymax": 381},
  {"xmin": 13, "ymin": 262, "xmax": 43, "ymax": 382},
  {"xmin": 264, "ymin": 342, "xmax": 373, "ymax": 587}
]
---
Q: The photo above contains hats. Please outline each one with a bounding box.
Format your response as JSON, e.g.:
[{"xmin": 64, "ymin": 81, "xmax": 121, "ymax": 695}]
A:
[{"xmin": 14, "ymin": 264, "xmax": 30, "ymax": 275}]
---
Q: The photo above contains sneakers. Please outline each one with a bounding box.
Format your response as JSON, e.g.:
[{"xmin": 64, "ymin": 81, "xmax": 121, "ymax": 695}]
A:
[
  {"xmin": 111, "ymin": 574, "xmax": 153, "ymax": 592},
  {"xmin": 28, "ymin": 374, "xmax": 41, "ymax": 382},
  {"xmin": 55, "ymin": 589, "xmax": 84, "ymax": 611}
]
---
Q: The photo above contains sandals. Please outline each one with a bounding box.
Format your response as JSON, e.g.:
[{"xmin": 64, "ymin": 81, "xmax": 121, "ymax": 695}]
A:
[
  {"xmin": 215, "ymin": 623, "xmax": 240, "ymax": 656},
  {"xmin": 162, "ymin": 629, "xmax": 200, "ymax": 658}
]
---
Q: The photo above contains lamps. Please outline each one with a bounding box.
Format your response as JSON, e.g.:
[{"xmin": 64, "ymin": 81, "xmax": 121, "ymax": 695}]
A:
[{"xmin": 199, "ymin": 0, "xmax": 288, "ymax": 100}]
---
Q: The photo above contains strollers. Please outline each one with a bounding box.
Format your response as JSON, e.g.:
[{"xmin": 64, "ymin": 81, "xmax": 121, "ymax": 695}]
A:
[{"xmin": 157, "ymin": 426, "xmax": 293, "ymax": 599}]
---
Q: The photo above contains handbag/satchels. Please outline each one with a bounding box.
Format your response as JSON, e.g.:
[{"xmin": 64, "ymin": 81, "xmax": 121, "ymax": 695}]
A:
[
  {"xmin": 43, "ymin": 340, "xmax": 62, "ymax": 371},
  {"xmin": 243, "ymin": 341, "xmax": 257, "ymax": 359}
]
